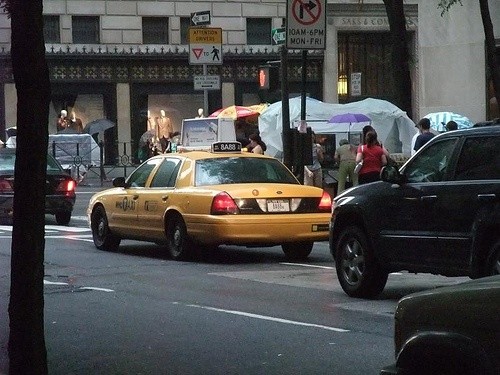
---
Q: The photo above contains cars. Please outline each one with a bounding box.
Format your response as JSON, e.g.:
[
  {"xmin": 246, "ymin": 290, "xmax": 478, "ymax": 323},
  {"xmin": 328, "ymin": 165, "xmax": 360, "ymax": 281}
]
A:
[
  {"xmin": 87, "ymin": 117, "xmax": 334, "ymax": 262},
  {"xmin": 0, "ymin": 147, "xmax": 77, "ymax": 226},
  {"xmin": 377, "ymin": 275, "xmax": 500, "ymax": 375}
]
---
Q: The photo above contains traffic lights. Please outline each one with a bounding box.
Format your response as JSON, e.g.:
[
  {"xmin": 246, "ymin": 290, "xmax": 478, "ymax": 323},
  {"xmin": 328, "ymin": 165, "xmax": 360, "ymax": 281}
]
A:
[{"xmin": 257, "ymin": 66, "xmax": 280, "ymax": 91}]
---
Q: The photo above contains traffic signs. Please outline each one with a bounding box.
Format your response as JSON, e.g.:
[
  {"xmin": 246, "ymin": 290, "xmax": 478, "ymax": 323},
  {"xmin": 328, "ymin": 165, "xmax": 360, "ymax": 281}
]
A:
[
  {"xmin": 271, "ymin": 27, "xmax": 286, "ymax": 46},
  {"xmin": 189, "ymin": 10, "xmax": 211, "ymax": 26}
]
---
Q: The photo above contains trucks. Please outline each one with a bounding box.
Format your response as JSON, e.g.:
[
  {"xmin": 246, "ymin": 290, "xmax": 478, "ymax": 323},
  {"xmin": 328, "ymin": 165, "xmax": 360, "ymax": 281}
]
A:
[{"xmin": 267, "ymin": 129, "xmax": 411, "ymax": 197}]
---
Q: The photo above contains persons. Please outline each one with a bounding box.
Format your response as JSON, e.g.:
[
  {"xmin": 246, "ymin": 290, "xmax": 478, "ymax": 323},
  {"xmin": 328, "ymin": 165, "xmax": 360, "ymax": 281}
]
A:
[
  {"xmin": 303, "ymin": 131, "xmax": 324, "ymax": 188},
  {"xmin": 414, "ymin": 118, "xmax": 436, "ymax": 152},
  {"xmin": 68, "ymin": 111, "xmax": 84, "ymax": 133},
  {"xmin": 57, "ymin": 109, "xmax": 70, "ymax": 132},
  {"xmin": 248, "ymin": 134, "xmax": 266, "ymax": 154},
  {"xmin": 446, "ymin": 121, "xmax": 458, "ymax": 131},
  {"xmin": 360, "ymin": 125, "xmax": 390, "ymax": 157},
  {"xmin": 195, "ymin": 107, "xmax": 207, "ymax": 118},
  {"xmin": 334, "ymin": 138, "xmax": 359, "ymax": 196},
  {"xmin": 410, "ymin": 120, "xmax": 422, "ymax": 156},
  {"xmin": 155, "ymin": 108, "xmax": 174, "ymax": 155},
  {"xmin": 355, "ymin": 130, "xmax": 387, "ymax": 186}
]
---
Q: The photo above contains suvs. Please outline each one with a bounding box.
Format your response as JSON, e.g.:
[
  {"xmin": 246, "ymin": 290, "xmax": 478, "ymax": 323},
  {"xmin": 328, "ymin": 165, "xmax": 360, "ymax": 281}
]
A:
[{"xmin": 329, "ymin": 125, "xmax": 500, "ymax": 299}]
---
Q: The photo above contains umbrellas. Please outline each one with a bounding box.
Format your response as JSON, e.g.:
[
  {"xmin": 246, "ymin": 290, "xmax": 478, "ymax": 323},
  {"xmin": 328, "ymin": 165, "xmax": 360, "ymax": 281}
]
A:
[
  {"xmin": 209, "ymin": 105, "xmax": 260, "ymax": 120},
  {"xmin": 329, "ymin": 113, "xmax": 371, "ymax": 124},
  {"xmin": 84, "ymin": 119, "xmax": 116, "ymax": 136}
]
